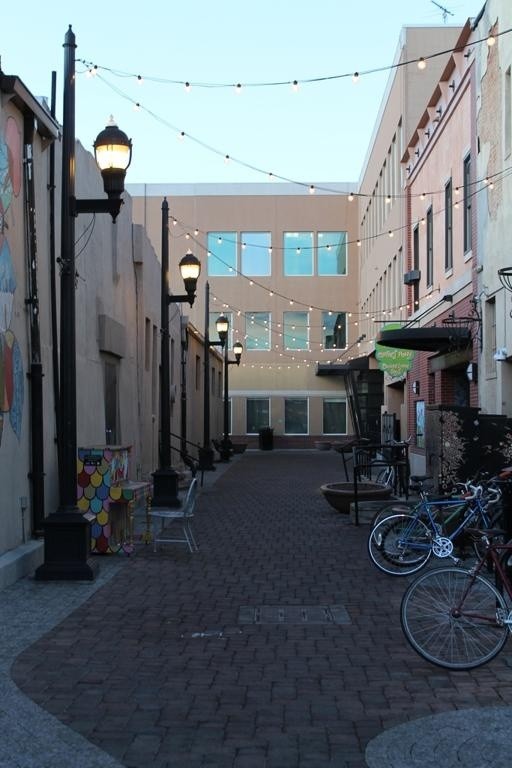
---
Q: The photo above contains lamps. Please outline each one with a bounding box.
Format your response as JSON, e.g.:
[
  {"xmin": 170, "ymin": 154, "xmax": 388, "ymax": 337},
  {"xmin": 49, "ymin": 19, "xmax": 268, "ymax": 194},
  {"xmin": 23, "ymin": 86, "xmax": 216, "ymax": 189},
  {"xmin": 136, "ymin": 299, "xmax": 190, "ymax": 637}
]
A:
[
  {"xmin": 467, "ymin": 363, "xmax": 478, "ymax": 381},
  {"xmin": 412, "ymin": 380, "xmax": 419, "ymax": 393}
]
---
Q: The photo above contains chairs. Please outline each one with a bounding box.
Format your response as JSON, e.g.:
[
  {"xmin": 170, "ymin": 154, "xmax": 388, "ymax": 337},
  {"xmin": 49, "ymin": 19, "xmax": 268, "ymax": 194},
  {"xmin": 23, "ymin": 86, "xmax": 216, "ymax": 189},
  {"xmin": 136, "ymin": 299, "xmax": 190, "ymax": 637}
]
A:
[{"xmin": 148, "ymin": 478, "xmax": 199, "ymax": 550}]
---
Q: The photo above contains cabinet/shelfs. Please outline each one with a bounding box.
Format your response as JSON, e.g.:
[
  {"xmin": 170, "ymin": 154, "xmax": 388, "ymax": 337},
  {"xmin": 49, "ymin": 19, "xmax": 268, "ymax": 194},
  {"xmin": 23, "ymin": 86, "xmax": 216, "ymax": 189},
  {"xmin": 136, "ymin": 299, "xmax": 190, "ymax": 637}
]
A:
[{"xmin": 78, "ymin": 444, "xmax": 150, "ymax": 554}]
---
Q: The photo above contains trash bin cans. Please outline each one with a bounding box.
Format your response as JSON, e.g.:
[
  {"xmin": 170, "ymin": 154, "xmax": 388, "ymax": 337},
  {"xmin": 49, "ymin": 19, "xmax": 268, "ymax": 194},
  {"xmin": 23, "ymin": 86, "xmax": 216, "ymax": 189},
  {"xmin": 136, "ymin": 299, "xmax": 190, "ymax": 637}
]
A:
[{"xmin": 258, "ymin": 425, "xmax": 274, "ymax": 451}]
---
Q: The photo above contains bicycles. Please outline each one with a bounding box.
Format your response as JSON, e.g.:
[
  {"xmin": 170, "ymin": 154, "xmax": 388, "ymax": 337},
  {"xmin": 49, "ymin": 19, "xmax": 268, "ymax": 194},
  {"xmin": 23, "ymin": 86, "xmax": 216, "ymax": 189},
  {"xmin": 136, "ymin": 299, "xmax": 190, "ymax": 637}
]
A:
[{"xmin": 369, "ymin": 430, "xmax": 512, "ymax": 669}]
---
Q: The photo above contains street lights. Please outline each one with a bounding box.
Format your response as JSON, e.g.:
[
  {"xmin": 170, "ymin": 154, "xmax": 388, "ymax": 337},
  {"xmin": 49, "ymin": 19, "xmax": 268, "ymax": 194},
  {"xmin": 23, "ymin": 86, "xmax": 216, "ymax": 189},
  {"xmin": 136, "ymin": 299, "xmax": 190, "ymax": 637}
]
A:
[
  {"xmin": 152, "ymin": 195, "xmax": 202, "ymax": 503},
  {"xmin": 30, "ymin": 22, "xmax": 130, "ymax": 578},
  {"xmin": 199, "ymin": 277, "xmax": 228, "ymax": 471},
  {"xmin": 221, "ymin": 330, "xmax": 243, "ymax": 462}
]
